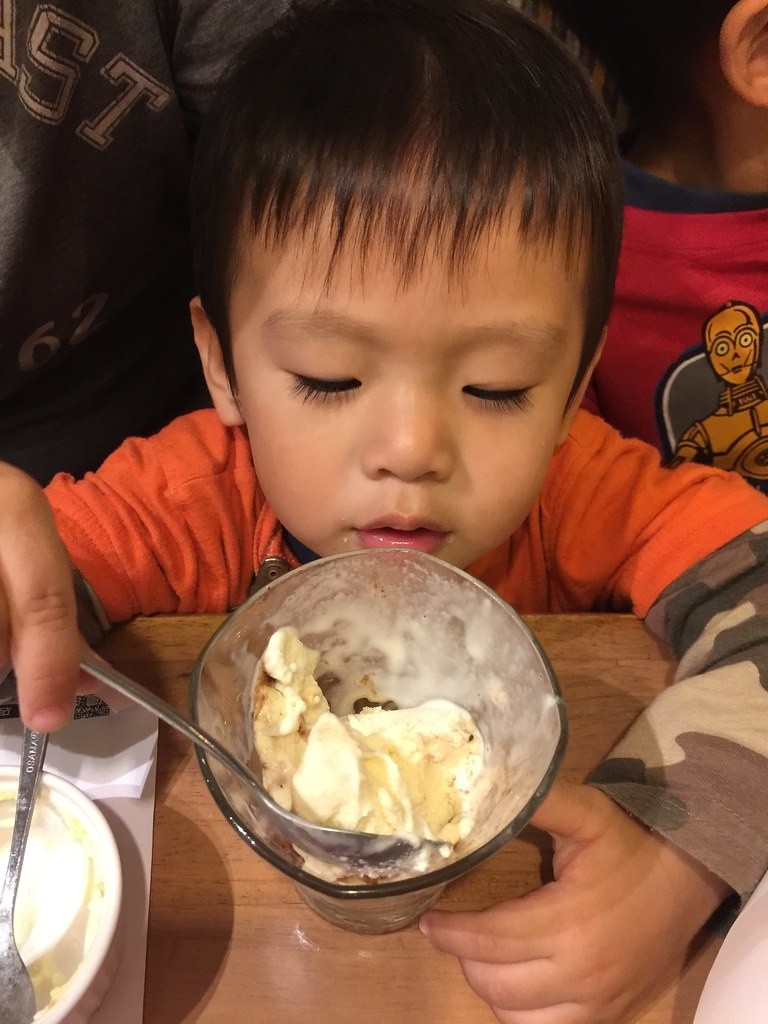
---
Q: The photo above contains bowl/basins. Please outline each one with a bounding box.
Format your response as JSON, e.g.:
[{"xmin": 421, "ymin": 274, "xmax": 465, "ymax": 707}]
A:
[{"xmin": 0, "ymin": 763, "xmax": 124, "ymax": 1024}]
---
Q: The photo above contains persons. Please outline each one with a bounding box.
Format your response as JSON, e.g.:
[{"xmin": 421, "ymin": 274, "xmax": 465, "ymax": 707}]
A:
[
  {"xmin": 0, "ymin": 0, "xmax": 768, "ymax": 1024},
  {"xmin": 564, "ymin": 0, "xmax": 767, "ymax": 495}
]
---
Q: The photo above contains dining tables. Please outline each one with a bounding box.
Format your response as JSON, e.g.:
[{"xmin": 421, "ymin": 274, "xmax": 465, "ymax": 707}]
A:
[{"xmin": 1, "ymin": 610, "xmax": 730, "ymax": 1022}]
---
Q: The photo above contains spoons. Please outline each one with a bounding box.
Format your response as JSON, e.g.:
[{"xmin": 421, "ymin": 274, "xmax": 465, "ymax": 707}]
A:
[
  {"xmin": 0, "ymin": 720, "xmax": 54, "ymax": 1022},
  {"xmin": 76, "ymin": 640, "xmax": 427, "ymax": 876}
]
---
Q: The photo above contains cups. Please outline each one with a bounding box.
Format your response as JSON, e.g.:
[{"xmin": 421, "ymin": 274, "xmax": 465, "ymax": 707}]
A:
[{"xmin": 185, "ymin": 546, "xmax": 564, "ymax": 938}]
